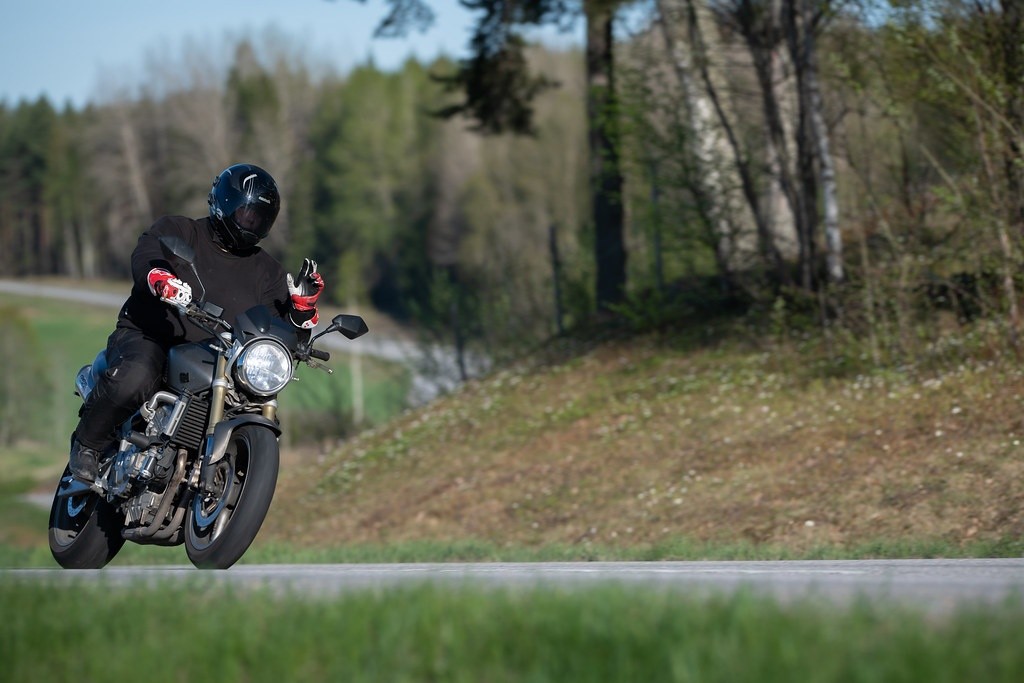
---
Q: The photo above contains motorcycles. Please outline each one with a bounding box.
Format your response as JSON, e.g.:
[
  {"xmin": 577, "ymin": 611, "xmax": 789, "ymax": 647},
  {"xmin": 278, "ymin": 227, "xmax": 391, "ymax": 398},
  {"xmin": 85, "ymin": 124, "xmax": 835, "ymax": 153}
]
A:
[{"xmin": 49, "ymin": 280, "xmax": 370, "ymax": 569}]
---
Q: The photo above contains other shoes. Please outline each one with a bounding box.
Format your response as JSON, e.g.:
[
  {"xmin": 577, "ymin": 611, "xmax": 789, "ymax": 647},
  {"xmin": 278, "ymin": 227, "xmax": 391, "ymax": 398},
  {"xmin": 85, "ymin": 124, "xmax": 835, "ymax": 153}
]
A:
[{"xmin": 69, "ymin": 436, "xmax": 97, "ymax": 483}]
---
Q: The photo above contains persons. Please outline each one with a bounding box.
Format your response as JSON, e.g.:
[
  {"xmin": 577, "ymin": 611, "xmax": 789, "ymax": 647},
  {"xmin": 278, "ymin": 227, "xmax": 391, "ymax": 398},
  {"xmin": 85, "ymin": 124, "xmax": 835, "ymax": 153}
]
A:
[{"xmin": 67, "ymin": 163, "xmax": 325, "ymax": 485}]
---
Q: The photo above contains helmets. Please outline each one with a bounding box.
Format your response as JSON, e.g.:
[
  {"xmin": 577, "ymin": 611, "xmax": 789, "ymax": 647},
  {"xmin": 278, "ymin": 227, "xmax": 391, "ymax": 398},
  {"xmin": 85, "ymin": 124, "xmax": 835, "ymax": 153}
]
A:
[{"xmin": 207, "ymin": 163, "xmax": 280, "ymax": 251}]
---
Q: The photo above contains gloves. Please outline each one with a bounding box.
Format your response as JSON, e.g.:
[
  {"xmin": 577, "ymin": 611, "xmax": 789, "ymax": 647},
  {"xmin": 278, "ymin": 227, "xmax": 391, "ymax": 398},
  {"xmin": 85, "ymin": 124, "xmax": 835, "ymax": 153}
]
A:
[
  {"xmin": 286, "ymin": 258, "xmax": 324, "ymax": 330},
  {"xmin": 147, "ymin": 265, "xmax": 192, "ymax": 307}
]
